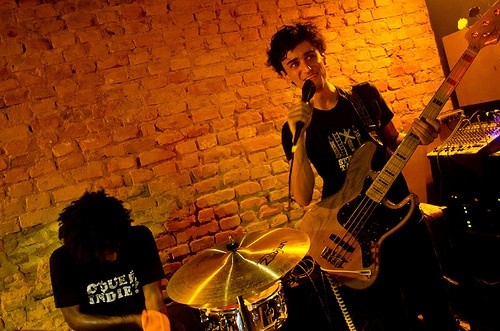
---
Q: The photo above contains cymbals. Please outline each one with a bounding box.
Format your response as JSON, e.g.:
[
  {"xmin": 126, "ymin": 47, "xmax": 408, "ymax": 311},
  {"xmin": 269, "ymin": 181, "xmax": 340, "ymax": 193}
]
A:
[{"xmin": 166, "ymin": 227, "xmax": 311, "ymax": 308}]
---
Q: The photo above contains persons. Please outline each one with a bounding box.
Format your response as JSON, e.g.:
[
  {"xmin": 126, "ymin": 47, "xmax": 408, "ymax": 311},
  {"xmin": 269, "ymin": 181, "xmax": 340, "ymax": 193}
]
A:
[
  {"xmin": 50, "ymin": 188, "xmax": 170, "ymax": 331},
  {"xmin": 264, "ymin": 22, "xmax": 459, "ymax": 331}
]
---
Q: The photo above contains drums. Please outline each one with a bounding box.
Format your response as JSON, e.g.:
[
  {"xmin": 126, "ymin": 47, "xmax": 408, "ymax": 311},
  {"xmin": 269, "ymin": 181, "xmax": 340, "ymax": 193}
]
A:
[{"xmin": 197, "ymin": 279, "xmax": 289, "ymax": 331}]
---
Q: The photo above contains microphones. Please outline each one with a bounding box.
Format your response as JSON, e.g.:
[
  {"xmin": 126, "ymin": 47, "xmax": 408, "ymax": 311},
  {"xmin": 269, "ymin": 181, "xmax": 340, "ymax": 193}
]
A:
[{"xmin": 294, "ymin": 80, "xmax": 316, "ymax": 145}]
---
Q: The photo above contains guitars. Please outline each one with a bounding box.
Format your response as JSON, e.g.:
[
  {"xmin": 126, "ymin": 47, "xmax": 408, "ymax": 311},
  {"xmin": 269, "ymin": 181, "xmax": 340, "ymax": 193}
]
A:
[{"xmin": 293, "ymin": 0, "xmax": 500, "ymax": 290}]
